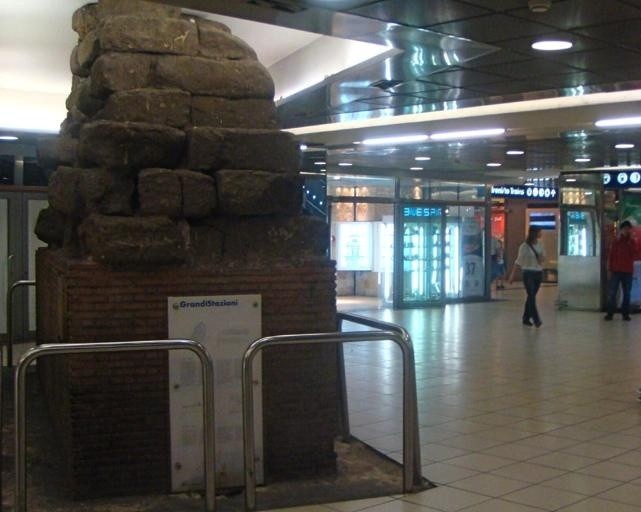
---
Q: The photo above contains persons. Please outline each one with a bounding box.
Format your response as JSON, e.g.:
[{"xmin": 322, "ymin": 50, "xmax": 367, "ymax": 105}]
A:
[
  {"xmin": 491, "ymin": 238, "xmax": 506, "ymax": 290},
  {"xmin": 603, "ymin": 220, "xmax": 638, "ymax": 321},
  {"xmin": 507, "ymin": 225, "xmax": 546, "ymax": 328}
]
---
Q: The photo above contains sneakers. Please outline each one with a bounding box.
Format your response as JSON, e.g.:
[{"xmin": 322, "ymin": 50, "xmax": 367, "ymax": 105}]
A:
[
  {"xmin": 604, "ymin": 313, "xmax": 631, "ymax": 321},
  {"xmin": 522, "ymin": 319, "xmax": 542, "ymax": 328}
]
